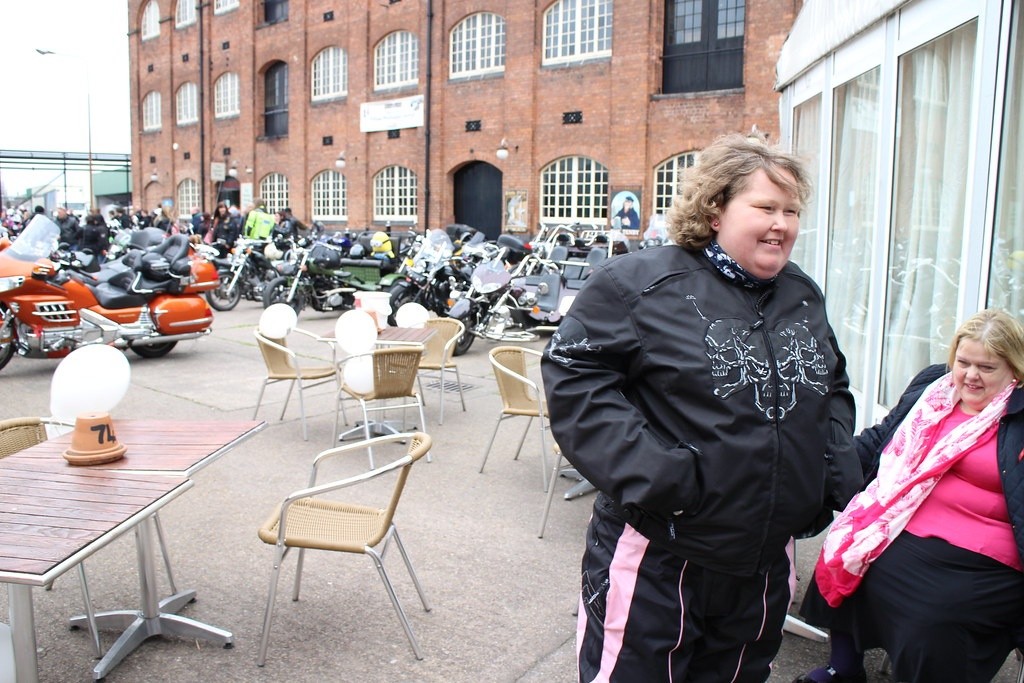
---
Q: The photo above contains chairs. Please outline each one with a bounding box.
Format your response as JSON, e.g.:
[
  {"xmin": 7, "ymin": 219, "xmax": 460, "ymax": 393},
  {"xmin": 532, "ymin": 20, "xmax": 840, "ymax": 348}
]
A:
[
  {"xmin": 252, "ymin": 317, "xmax": 564, "ymax": 668},
  {"xmin": 0, "ymin": 417, "xmax": 179, "ymax": 661}
]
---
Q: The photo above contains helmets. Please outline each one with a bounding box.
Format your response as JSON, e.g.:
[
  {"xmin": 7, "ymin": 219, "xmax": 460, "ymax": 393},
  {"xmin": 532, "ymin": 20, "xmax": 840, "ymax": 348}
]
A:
[
  {"xmin": 350, "ymin": 243, "xmax": 365, "ymax": 259},
  {"xmin": 142, "ymin": 252, "xmax": 170, "ymax": 282}
]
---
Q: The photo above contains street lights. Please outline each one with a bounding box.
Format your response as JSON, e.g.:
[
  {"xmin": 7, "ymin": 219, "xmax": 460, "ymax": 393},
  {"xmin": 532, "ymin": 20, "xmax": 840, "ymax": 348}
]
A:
[{"xmin": 36, "ymin": 48, "xmax": 95, "ymax": 215}]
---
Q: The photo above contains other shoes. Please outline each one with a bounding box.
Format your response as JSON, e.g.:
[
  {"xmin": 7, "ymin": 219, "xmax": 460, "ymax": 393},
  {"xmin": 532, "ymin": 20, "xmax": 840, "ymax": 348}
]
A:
[{"xmin": 791, "ymin": 664, "xmax": 867, "ymax": 683}]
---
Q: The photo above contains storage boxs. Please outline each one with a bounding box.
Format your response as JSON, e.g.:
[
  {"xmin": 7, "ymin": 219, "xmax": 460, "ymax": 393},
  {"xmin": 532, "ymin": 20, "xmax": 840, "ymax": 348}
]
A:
[
  {"xmin": 444, "ymin": 224, "xmax": 476, "ymax": 246},
  {"xmin": 497, "ymin": 234, "xmax": 533, "ymax": 264},
  {"xmin": 127, "ymin": 228, "xmax": 170, "ymax": 250}
]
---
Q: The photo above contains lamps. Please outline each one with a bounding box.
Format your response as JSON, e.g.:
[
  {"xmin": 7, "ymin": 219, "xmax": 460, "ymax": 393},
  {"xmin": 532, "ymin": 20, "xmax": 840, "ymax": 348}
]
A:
[
  {"xmin": 228, "ymin": 160, "xmax": 252, "ymax": 179},
  {"xmin": 151, "ymin": 168, "xmax": 170, "ymax": 182},
  {"xmin": 495, "ymin": 137, "xmax": 519, "ymax": 160},
  {"xmin": 335, "ymin": 149, "xmax": 346, "ymax": 169}
]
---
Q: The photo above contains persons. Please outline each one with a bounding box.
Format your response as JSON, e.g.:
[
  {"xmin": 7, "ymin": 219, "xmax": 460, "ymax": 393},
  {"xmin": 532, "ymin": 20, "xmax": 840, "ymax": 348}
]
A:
[
  {"xmin": 613, "ymin": 196, "xmax": 639, "ymax": 229},
  {"xmin": 0, "ymin": 200, "xmax": 245, "ymax": 272},
  {"xmin": 540, "ymin": 129, "xmax": 857, "ymax": 683},
  {"xmin": 283, "ymin": 208, "xmax": 309, "ymax": 239},
  {"xmin": 790, "ymin": 310, "xmax": 1024, "ymax": 683},
  {"xmin": 273, "ymin": 211, "xmax": 297, "ymax": 242},
  {"xmin": 242, "ymin": 197, "xmax": 276, "ymax": 247}
]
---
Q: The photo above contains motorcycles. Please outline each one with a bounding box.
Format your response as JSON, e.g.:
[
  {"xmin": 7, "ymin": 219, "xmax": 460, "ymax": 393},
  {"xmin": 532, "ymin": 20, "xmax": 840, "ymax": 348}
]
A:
[{"xmin": 0, "ymin": 208, "xmax": 685, "ymax": 378}]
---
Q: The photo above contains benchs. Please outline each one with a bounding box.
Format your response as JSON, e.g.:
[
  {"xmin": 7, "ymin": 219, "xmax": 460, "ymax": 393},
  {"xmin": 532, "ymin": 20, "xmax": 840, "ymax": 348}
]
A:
[
  {"xmin": 525, "ymin": 246, "xmax": 607, "ymax": 290},
  {"xmin": 338, "ymin": 235, "xmax": 401, "ymax": 268}
]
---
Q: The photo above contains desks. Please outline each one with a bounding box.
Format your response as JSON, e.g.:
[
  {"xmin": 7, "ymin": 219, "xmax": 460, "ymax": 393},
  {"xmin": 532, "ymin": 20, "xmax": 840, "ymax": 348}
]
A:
[
  {"xmin": 316, "ymin": 326, "xmax": 438, "ymax": 445},
  {"xmin": 0, "ymin": 460, "xmax": 195, "ymax": 683},
  {"xmin": 5, "ymin": 418, "xmax": 269, "ymax": 683}
]
